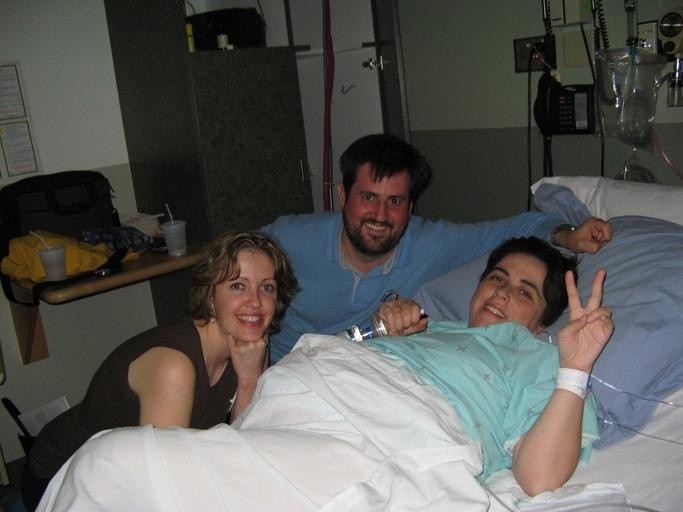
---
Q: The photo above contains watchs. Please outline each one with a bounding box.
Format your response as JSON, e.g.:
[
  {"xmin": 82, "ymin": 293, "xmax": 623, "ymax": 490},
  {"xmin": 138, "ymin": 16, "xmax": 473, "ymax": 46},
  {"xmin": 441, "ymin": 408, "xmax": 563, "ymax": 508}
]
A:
[{"xmin": 549, "ymin": 224, "xmax": 575, "ymax": 246}]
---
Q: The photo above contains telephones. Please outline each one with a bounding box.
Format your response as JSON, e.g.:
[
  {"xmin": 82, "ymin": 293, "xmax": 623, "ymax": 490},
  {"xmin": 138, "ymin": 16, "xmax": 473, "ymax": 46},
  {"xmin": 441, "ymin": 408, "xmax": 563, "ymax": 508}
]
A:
[{"xmin": 533, "ymin": 72, "xmax": 596, "ymax": 136}]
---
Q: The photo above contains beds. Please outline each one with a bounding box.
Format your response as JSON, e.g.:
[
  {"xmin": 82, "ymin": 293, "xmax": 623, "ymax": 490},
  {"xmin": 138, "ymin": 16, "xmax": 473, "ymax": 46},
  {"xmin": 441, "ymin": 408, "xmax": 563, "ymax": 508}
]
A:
[{"xmin": 45, "ymin": 175, "xmax": 683, "ymax": 511}]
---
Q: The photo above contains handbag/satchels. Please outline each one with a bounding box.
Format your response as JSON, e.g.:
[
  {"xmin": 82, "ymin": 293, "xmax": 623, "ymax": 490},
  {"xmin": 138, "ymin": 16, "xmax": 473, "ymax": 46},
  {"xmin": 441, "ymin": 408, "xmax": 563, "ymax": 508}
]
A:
[
  {"xmin": 79, "ymin": 221, "xmax": 158, "ymax": 250},
  {"xmin": 0, "ymin": 229, "xmax": 108, "ymax": 282}
]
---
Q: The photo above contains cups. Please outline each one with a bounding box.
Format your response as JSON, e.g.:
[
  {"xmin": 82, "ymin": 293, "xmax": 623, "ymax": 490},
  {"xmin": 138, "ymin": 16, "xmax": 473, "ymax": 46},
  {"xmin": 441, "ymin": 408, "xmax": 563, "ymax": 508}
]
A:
[
  {"xmin": 161, "ymin": 222, "xmax": 188, "ymax": 257},
  {"xmin": 39, "ymin": 247, "xmax": 67, "ymax": 281}
]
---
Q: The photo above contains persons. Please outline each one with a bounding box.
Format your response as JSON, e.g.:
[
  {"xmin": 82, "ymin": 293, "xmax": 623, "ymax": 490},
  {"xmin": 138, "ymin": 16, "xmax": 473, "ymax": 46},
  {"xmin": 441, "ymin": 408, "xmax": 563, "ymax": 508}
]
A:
[
  {"xmin": 35, "ymin": 233, "xmax": 615, "ymax": 512},
  {"xmin": 246, "ymin": 134, "xmax": 613, "ymax": 372},
  {"xmin": 20, "ymin": 229, "xmax": 303, "ymax": 512}
]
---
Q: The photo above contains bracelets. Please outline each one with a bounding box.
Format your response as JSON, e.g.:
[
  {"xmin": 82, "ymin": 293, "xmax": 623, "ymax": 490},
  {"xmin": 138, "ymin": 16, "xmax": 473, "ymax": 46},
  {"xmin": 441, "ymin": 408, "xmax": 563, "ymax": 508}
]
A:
[{"xmin": 555, "ymin": 366, "xmax": 589, "ymax": 401}]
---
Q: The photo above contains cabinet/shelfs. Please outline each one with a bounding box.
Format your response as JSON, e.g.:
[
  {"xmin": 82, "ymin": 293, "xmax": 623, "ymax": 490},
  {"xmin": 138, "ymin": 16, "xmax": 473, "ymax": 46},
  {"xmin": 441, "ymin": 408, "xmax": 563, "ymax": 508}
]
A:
[{"xmin": 106, "ymin": 1, "xmax": 317, "ymax": 241}]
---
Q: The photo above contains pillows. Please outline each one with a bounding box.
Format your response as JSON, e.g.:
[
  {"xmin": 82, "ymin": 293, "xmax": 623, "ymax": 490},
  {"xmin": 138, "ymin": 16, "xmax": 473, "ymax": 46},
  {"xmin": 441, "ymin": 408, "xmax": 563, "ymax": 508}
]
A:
[{"xmin": 409, "ymin": 216, "xmax": 683, "ymax": 449}]
